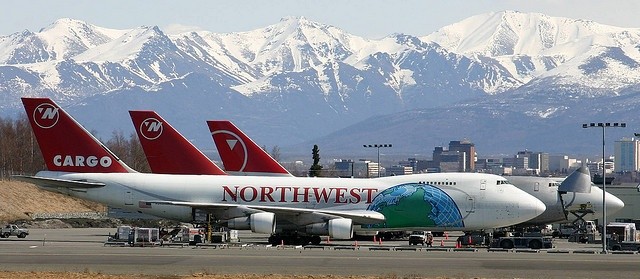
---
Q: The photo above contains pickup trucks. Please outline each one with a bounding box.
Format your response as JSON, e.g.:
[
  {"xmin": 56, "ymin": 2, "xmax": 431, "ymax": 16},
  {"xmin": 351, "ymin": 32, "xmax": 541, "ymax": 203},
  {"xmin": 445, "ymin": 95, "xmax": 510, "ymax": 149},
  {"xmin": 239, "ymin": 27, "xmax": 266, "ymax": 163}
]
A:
[{"xmin": 0, "ymin": 224, "xmax": 29, "ymax": 238}]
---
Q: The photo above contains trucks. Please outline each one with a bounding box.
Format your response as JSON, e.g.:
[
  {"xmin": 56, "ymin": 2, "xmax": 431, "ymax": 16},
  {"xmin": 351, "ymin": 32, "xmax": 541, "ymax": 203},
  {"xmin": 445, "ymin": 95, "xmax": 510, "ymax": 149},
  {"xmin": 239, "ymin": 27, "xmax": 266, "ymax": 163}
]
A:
[
  {"xmin": 552, "ymin": 220, "xmax": 597, "ymax": 239},
  {"xmin": 408, "ymin": 230, "xmax": 431, "ymax": 247}
]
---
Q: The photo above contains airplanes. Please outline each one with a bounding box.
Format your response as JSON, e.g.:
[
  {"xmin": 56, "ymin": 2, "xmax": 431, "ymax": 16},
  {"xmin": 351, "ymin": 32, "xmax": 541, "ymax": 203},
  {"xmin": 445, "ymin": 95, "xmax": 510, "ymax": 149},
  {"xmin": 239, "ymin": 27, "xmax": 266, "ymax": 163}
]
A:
[
  {"xmin": 205, "ymin": 119, "xmax": 626, "ymax": 237},
  {"xmin": 10, "ymin": 98, "xmax": 546, "ymax": 245}
]
